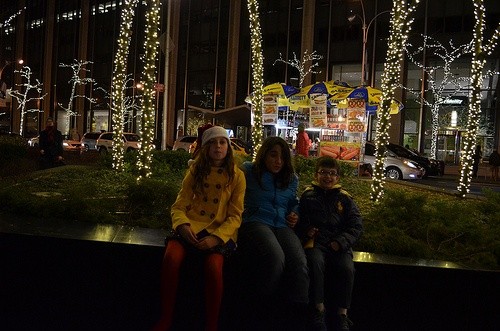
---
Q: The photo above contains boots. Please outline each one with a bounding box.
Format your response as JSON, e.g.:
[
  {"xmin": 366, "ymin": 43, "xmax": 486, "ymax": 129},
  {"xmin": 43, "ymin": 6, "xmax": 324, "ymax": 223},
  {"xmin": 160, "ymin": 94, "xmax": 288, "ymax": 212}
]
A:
[{"xmin": 151, "ymin": 264, "xmax": 180, "ymax": 331}]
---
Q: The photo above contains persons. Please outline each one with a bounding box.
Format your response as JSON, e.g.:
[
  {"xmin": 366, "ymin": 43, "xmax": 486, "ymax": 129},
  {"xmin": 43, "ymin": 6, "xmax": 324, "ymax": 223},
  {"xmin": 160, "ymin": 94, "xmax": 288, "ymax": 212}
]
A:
[
  {"xmin": 153, "ymin": 125, "xmax": 246, "ymax": 331},
  {"xmin": 239, "ymin": 137, "xmax": 309, "ymax": 331},
  {"xmin": 298, "ymin": 156, "xmax": 364, "ymax": 330},
  {"xmin": 294, "ymin": 125, "xmax": 311, "ymax": 159},
  {"xmin": 39, "ymin": 118, "xmax": 64, "ymax": 169}
]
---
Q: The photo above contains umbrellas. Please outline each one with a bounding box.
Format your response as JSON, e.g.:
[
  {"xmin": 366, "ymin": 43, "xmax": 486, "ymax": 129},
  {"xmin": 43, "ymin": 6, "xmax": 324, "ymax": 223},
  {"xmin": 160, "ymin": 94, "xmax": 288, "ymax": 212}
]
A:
[
  {"xmin": 325, "ymin": 83, "xmax": 404, "ymax": 140},
  {"xmin": 244, "ymin": 82, "xmax": 300, "ymax": 138},
  {"xmin": 287, "ymin": 79, "xmax": 351, "ymax": 141}
]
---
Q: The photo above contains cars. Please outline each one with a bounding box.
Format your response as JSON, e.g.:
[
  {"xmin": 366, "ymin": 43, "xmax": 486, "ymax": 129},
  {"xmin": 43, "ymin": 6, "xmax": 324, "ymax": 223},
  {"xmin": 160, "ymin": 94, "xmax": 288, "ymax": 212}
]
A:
[
  {"xmin": 27, "ymin": 134, "xmax": 81, "ymax": 152},
  {"xmin": 387, "ymin": 142, "xmax": 446, "ymax": 177},
  {"xmin": 95, "ymin": 131, "xmax": 157, "ymax": 155},
  {"xmin": 362, "ymin": 140, "xmax": 427, "ymax": 180},
  {"xmin": 79, "ymin": 133, "xmax": 101, "ymax": 153},
  {"xmin": 172, "ymin": 135, "xmax": 198, "ymax": 155},
  {"xmin": 188, "ymin": 139, "xmax": 251, "ymax": 157}
]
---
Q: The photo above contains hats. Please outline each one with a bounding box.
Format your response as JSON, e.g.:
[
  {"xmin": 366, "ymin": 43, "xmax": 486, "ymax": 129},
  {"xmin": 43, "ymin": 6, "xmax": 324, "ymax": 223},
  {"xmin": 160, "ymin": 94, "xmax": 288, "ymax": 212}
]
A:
[{"xmin": 201, "ymin": 126, "xmax": 230, "ymax": 146}]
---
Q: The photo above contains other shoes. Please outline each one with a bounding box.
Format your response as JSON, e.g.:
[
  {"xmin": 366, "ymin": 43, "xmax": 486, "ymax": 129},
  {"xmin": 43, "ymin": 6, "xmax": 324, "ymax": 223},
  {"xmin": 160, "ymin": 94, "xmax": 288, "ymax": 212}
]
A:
[
  {"xmin": 311, "ymin": 307, "xmax": 327, "ymax": 331},
  {"xmin": 204, "ymin": 274, "xmax": 224, "ymax": 331},
  {"xmin": 335, "ymin": 313, "xmax": 351, "ymax": 331}
]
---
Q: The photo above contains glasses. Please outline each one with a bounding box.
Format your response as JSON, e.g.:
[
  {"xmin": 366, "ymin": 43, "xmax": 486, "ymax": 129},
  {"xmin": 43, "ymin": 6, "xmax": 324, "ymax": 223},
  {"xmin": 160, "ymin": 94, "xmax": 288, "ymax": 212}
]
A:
[{"xmin": 317, "ymin": 169, "xmax": 336, "ymax": 175}]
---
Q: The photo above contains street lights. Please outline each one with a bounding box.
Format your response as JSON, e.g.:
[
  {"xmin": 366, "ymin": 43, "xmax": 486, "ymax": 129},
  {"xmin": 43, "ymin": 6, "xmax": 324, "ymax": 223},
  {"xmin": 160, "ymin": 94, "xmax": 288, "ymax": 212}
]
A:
[
  {"xmin": 9, "ymin": 58, "xmax": 24, "ymax": 133},
  {"xmin": 346, "ymin": 9, "xmax": 400, "ymax": 87}
]
